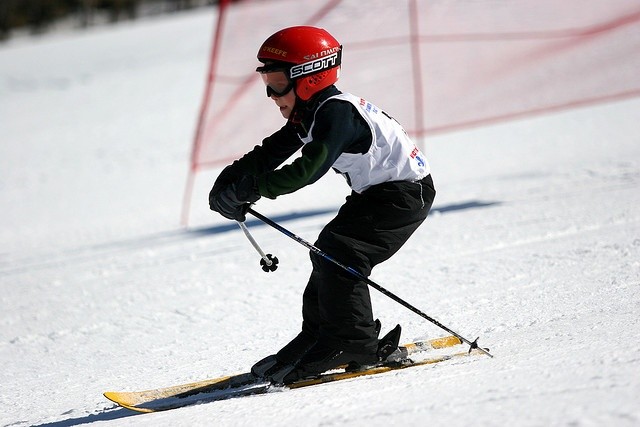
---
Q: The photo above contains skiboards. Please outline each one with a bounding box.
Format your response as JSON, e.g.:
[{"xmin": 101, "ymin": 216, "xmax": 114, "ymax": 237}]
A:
[{"xmin": 104, "ymin": 335, "xmax": 490, "ymax": 414}]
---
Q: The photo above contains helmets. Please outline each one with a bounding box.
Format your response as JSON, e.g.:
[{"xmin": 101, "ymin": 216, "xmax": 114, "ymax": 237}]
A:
[{"xmin": 257, "ymin": 25, "xmax": 341, "ymax": 100}]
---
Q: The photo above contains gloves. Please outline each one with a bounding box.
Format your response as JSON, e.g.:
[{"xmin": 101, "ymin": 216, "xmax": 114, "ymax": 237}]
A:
[
  {"xmin": 209, "ymin": 165, "xmax": 245, "ymax": 210},
  {"xmin": 211, "ymin": 174, "xmax": 259, "ymax": 221}
]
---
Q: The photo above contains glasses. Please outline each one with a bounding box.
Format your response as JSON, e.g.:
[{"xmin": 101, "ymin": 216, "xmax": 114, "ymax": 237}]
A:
[{"xmin": 257, "ymin": 51, "xmax": 341, "ymax": 96}]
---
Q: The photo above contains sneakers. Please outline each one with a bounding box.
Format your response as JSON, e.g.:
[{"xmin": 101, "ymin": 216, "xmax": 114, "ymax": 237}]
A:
[
  {"xmin": 276, "ymin": 329, "xmax": 316, "ymax": 365},
  {"xmin": 296, "ymin": 338, "xmax": 379, "ymax": 376}
]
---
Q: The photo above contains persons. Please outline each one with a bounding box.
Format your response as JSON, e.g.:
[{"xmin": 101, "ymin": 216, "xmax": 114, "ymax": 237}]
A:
[{"xmin": 208, "ymin": 26, "xmax": 436, "ymax": 385}]
